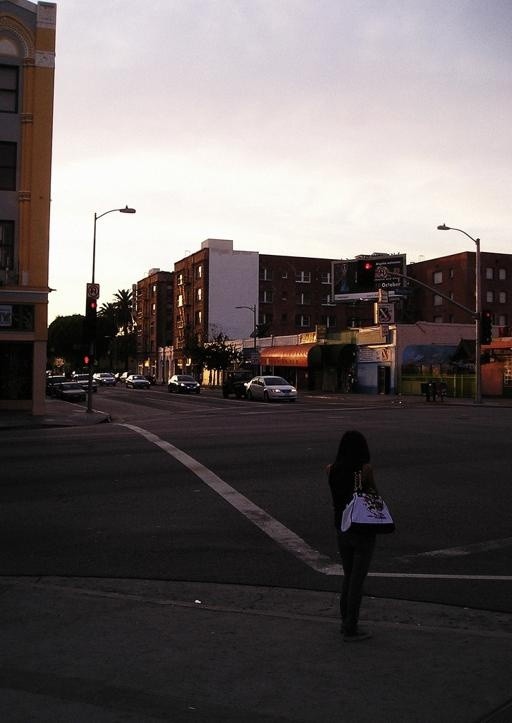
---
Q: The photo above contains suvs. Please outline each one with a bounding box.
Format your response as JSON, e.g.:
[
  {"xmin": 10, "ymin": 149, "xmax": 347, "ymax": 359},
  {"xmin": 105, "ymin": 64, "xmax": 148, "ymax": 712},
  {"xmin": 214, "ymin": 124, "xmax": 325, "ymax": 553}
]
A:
[{"xmin": 221, "ymin": 372, "xmax": 254, "ymax": 400}]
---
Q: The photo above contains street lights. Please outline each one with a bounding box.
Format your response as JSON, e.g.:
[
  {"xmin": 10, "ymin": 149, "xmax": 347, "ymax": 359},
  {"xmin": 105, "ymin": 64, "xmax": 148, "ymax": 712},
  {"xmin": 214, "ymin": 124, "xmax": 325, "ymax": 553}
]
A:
[
  {"xmin": 86, "ymin": 206, "xmax": 135, "ymax": 413},
  {"xmin": 437, "ymin": 224, "xmax": 482, "ymax": 404},
  {"xmin": 235, "ymin": 304, "xmax": 256, "ymax": 348}
]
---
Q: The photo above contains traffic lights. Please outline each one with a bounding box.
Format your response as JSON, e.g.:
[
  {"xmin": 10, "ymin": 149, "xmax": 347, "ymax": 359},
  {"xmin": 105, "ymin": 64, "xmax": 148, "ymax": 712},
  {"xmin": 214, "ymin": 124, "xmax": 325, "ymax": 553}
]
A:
[
  {"xmin": 83, "ymin": 355, "xmax": 89, "ymax": 364},
  {"xmin": 86, "ymin": 298, "xmax": 97, "ymax": 319},
  {"xmin": 482, "ymin": 311, "xmax": 492, "ymax": 345},
  {"xmin": 357, "ymin": 260, "xmax": 376, "ymax": 288}
]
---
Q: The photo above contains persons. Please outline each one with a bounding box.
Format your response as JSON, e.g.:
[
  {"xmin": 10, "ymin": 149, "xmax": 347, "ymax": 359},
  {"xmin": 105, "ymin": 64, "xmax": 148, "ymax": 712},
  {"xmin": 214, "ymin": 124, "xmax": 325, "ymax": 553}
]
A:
[{"xmin": 327, "ymin": 431, "xmax": 378, "ymax": 643}]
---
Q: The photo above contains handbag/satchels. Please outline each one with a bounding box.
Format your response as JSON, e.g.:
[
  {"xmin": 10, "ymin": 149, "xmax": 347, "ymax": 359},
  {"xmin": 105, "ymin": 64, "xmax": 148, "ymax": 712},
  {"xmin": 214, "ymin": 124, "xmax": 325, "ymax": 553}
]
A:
[{"xmin": 340, "ymin": 491, "xmax": 396, "ymax": 534}]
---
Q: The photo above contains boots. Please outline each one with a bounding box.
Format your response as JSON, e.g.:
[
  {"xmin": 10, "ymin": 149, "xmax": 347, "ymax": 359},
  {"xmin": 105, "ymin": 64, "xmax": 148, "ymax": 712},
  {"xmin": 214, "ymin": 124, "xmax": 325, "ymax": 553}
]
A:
[{"xmin": 338, "ymin": 597, "xmax": 371, "ymax": 640}]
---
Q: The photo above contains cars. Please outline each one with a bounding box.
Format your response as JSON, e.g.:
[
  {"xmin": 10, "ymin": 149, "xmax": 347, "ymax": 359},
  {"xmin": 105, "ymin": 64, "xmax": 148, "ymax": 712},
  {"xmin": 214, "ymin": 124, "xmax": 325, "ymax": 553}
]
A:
[
  {"xmin": 168, "ymin": 374, "xmax": 200, "ymax": 394},
  {"xmin": 46, "ymin": 374, "xmax": 97, "ymax": 403},
  {"xmin": 119, "ymin": 372, "xmax": 151, "ymax": 389},
  {"xmin": 246, "ymin": 375, "xmax": 298, "ymax": 403},
  {"xmin": 93, "ymin": 373, "xmax": 116, "ymax": 387}
]
